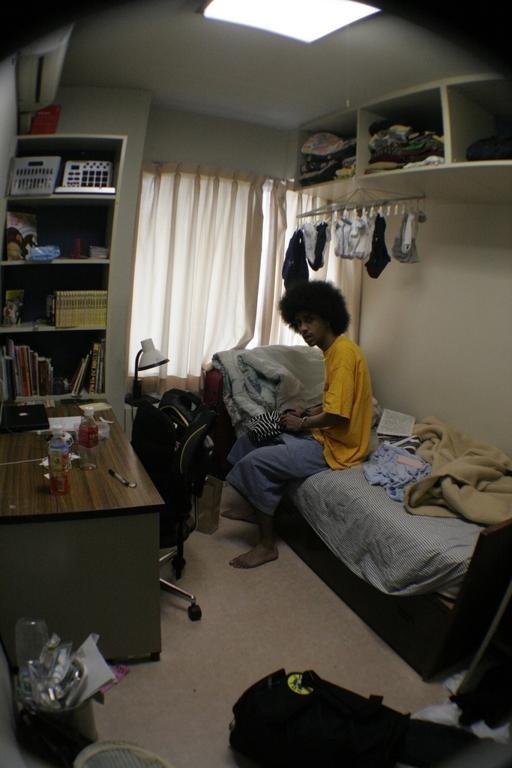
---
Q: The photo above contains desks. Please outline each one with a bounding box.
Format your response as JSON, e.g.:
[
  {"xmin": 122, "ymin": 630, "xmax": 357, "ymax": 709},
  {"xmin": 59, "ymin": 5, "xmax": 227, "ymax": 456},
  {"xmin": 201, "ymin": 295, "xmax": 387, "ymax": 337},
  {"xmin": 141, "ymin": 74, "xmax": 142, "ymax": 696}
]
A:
[{"xmin": 0, "ymin": 397, "xmax": 166, "ymax": 665}]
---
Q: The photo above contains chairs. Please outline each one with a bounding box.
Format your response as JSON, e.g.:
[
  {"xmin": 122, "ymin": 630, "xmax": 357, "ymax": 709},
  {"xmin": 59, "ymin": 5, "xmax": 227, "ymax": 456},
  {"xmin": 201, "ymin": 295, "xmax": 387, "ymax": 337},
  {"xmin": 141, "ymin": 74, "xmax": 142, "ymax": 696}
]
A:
[{"xmin": 132, "ymin": 402, "xmax": 216, "ymax": 623}]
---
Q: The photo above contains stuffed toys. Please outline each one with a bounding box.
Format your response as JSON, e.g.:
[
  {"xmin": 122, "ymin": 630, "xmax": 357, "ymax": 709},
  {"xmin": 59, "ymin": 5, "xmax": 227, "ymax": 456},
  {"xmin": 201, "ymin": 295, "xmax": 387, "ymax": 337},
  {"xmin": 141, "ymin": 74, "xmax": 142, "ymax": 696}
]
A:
[{"xmin": 7, "ymin": 211, "xmax": 38, "ymax": 261}]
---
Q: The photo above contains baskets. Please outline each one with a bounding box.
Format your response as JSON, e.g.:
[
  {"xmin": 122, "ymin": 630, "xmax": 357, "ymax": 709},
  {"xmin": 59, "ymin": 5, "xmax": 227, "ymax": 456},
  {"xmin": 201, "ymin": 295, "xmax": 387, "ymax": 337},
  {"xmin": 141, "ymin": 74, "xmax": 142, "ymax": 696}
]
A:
[
  {"xmin": 62, "ymin": 161, "xmax": 113, "ymax": 187},
  {"xmin": 10, "ymin": 156, "xmax": 61, "ymax": 195},
  {"xmin": 55, "ymin": 290, "xmax": 107, "ymax": 328}
]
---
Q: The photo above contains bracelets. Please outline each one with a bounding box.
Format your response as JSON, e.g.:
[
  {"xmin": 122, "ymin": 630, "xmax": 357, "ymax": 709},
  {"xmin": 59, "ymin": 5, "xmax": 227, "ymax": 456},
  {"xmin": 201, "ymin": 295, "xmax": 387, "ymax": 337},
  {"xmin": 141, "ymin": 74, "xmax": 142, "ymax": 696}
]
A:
[{"xmin": 298, "ymin": 416, "xmax": 307, "ymax": 430}]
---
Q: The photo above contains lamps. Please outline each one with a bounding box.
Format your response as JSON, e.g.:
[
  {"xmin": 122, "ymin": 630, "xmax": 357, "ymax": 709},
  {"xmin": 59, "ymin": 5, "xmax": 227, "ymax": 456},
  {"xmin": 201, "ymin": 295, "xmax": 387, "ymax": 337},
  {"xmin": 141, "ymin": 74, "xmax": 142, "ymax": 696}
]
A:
[{"xmin": 123, "ymin": 337, "xmax": 172, "ymax": 407}]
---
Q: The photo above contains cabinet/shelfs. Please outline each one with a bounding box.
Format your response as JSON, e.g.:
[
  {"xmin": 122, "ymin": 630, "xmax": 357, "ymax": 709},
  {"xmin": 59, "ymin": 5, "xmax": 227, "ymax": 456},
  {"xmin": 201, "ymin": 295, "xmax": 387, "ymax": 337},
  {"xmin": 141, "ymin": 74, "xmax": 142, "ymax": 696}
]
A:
[
  {"xmin": 0, "ymin": 132, "xmax": 128, "ymax": 402},
  {"xmin": 294, "ymin": 73, "xmax": 511, "ymax": 204}
]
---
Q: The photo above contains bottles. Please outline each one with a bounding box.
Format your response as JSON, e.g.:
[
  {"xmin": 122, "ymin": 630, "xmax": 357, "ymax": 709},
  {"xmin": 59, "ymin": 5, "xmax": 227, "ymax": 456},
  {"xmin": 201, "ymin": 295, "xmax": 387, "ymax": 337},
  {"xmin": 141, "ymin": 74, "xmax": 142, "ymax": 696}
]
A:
[
  {"xmin": 45, "ymin": 425, "xmax": 71, "ymax": 497},
  {"xmin": 79, "ymin": 407, "xmax": 100, "ymax": 471}
]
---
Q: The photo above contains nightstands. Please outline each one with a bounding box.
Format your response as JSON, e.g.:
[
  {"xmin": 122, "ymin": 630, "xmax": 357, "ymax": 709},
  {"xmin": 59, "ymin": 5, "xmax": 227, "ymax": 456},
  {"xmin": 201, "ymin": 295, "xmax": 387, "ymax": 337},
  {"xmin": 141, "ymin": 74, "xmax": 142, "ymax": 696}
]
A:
[{"xmin": 124, "ymin": 390, "xmax": 164, "ymax": 427}]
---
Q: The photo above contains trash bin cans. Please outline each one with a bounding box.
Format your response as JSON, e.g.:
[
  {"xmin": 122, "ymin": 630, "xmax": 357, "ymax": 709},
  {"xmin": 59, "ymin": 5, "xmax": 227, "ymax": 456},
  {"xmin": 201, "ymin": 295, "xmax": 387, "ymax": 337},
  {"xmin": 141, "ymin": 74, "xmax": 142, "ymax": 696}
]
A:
[{"xmin": 13, "ymin": 674, "xmax": 99, "ymax": 767}]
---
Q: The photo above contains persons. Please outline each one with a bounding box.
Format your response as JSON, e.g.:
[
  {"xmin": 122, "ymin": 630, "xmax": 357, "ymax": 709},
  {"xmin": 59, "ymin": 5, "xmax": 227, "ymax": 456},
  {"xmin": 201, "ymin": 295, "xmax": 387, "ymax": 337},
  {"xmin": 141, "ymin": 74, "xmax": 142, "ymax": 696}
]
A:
[{"xmin": 220, "ymin": 280, "xmax": 372, "ymax": 569}]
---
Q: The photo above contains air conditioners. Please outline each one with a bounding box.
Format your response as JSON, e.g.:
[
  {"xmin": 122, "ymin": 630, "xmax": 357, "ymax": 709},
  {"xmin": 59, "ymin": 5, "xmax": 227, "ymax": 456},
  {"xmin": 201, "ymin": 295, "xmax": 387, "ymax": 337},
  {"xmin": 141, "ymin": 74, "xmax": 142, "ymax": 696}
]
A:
[{"xmin": 10, "ymin": 22, "xmax": 75, "ymax": 111}]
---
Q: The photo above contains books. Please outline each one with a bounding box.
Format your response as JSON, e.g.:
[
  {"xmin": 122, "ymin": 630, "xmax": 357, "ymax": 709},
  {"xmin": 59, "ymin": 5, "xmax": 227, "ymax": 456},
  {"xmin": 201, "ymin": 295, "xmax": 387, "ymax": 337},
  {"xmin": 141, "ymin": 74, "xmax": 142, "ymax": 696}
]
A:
[
  {"xmin": 376, "ymin": 409, "xmax": 416, "ymax": 442},
  {"xmin": 0, "ymin": 291, "xmax": 107, "ymax": 402}
]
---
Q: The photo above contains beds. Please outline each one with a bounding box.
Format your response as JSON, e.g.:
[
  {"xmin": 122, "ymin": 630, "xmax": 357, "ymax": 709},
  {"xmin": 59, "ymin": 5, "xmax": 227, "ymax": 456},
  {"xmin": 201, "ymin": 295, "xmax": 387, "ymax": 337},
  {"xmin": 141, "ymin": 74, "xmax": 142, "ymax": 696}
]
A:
[{"xmin": 202, "ymin": 345, "xmax": 512, "ymax": 680}]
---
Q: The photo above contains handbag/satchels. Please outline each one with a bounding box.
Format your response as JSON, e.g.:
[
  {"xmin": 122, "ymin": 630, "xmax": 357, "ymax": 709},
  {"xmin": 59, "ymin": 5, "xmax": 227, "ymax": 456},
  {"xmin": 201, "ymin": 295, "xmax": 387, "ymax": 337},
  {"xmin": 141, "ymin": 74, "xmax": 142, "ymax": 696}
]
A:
[
  {"xmin": 227, "ymin": 669, "xmax": 410, "ymax": 768},
  {"xmin": 193, "ymin": 475, "xmax": 223, "ymax": 534},
  {"xmin": 247, "ymin": 411, "xmax": 285, "ymax": 448}
]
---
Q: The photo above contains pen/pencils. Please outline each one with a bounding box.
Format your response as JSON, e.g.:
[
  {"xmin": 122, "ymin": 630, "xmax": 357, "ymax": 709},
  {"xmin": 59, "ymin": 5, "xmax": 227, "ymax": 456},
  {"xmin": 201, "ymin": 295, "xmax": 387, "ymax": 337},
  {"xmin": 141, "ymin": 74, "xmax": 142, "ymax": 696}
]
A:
[{"xmin": 108, "ymin": 468, "xmax": 130, "ymax": 487}]
